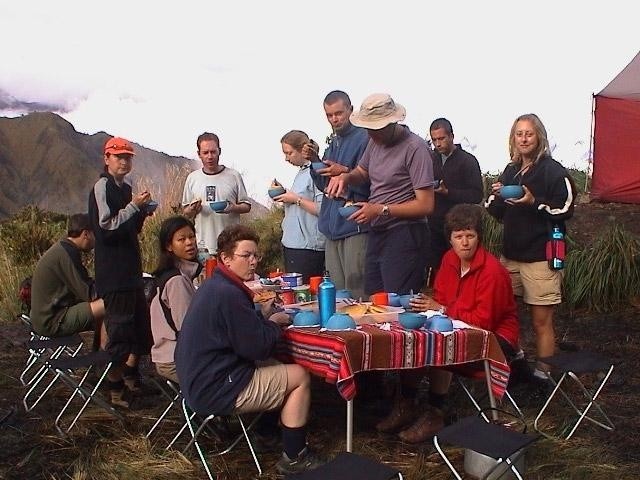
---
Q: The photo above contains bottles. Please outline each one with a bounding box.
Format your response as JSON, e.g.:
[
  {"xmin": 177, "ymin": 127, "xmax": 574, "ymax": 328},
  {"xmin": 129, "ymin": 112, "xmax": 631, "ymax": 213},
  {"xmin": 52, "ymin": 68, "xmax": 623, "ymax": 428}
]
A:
[
  {"xmin": 256, "ymin": 269, "xmax": 324, "ymax": 314},
  {"xmin": 324, "ymin": 188, "xmax": 348, "ymax": 201},
  {"xmin": 196, "ymin": 240, "xmax": 210, "ymax": 266},
  {"xmin": 318, "ymin": 270, "xmax": 336, "ymax": 328},
  {"xmin": 549, "ymin": 224, "xmax": 565, "ymax": 268}
]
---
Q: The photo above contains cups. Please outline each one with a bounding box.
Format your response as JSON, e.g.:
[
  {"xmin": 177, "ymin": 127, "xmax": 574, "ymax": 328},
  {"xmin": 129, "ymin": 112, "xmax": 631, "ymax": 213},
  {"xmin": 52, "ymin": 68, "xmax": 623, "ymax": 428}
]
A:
[{"xmin": 206, "ymin": 257, "xmax": 216, "ymax": 279}]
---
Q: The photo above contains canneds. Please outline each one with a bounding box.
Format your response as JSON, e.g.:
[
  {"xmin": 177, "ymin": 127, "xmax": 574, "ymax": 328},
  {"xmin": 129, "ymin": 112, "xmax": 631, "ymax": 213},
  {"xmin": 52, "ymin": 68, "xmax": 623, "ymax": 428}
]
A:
[
  {"xmin": 282, "ymin": 273, "xmax": 303, "ymax": 286},
  {"xmin": 293, "ymin": 287, "xmax": 312, "ymax": 304}
]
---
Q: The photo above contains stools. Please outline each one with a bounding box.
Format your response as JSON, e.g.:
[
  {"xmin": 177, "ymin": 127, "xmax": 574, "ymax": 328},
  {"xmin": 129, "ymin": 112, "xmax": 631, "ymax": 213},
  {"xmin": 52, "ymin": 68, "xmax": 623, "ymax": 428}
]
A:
[
  {"xmin": 532, "ymin": 352, "xmax": 619, "ymax": 443},
  {"xmin": 137, "ymin": 373, "xmax": 265, "ymax": 479},
  {"xmin": 281, "ymin": 451, "xmax": 404, "ymax": 479},
  {"xmin": 17, "ymin": 315, "xmax": 131, "ymax": 438},
  {"xmin": 435, "ymin": 418, "xmax": 543, "ymax": 479},
  {"xmin": 448, "ymin": 350, "xmax": 525, "ymax": 422}
]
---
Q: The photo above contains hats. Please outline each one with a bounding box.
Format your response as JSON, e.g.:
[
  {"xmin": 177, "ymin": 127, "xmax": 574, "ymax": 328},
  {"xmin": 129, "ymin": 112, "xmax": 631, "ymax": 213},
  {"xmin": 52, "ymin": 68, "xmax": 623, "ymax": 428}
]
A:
[
  {"xmin": 104, "ymin": 137, "xmax": 136, "ymax": 155},
  {"xmin": 349, "ymin": 93, "xmax": 406, "ymax": 130}
]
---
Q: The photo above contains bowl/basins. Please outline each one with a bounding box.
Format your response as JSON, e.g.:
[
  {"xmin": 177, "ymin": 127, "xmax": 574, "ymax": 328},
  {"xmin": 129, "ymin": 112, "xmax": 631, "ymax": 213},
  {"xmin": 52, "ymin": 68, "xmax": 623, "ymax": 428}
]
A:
[
  {"xmin": 292, "ymin": 309, "xmax": 320, "ymax": 328},
  {"xmin": 432, "ymin": 180, "xmax": 440, "ymax": 189},
  {"xmin": 430, "ymin": 315, "xmax": 455, "ymax": 332},
  {"xmin": 397, "ymin": 313, "xmax": 427, "ymax": 329},
  {"xmin": 400, "ymin": 295, "xmax": 420, "ymax": 308},
  {"xmin": 335, "ymin": 290, "xmax": 351, "ymax": 298},
  {"xmin": 373, "ymin": 292, "xmax": 401, "ymax": 306},
  {"xmin": 422, "ymin": 315, "xmax": 440, "ymax": 329},
  {"xmin": 325, "ymin": 311, "xmax": 357, "ymax": 331},
  {"xmin": 268, "ymin": 188, "xmax": 286, "ymax": 200},
  {"xmin": 312, "ymin": 162, "xmax": 327, "ymax": 170},
  {"xmin": 336, "ymin": 302, "xmax": 405, "ymax": 326},
  {"xmin": 209, "ymin": 200, "xmax": 227, "ymax": 213},
  {"xmin": 499, "ymin": 186, "xmax": 524, "ymax": 202},
  {"xmin": 146, "ymin": 201, "xmax": 158, "ymax": 211},
  {"xmin": 337, "ymin": 205, "xmax": 362, "ymax": 219}
]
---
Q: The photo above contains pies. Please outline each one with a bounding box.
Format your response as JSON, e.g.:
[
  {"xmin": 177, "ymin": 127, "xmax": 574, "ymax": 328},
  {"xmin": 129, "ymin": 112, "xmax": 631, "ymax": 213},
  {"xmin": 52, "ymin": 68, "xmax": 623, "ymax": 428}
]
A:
[{"xmin": 337, "ymin": 304, "xmax": 389, "ymax": 322}]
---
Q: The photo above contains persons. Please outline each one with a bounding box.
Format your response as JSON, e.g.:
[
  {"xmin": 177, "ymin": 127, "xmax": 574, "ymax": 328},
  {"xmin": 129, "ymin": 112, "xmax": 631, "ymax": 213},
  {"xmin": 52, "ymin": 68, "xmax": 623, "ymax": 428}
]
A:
[
  {"xmin": 86, "ymin": 135, "xmax": 155, "ymax": 410},
  {"xmin": 272, "ymin": 131, "xmax": 325, "ymax": 283},
  {"xmin": 26, "ymin": 212, "xmax": 111, "ymax": 378},
  {"xmin": 430, "ymin": 118, "xmax": 483, "ymax": 287},
  {"xmin": 484, "ymin": 113, "xmax": 576, "ymax": 398},
  {"xmin": 375, "ymin": 202, "xmax": 520, "ymax": 444},
  {"xmin": 146, "ymin": 216, "xmax": 200, "ymax": 386},
  {"xmin": 325, "ymin": 93, "xmax": 435, "ymax": 297},
  {"xmin": 174, "ymin": 223, "xmax": 337, "ymax": 476},
  {"xmin": 180, "ymin": 130, "xmax": 253, "ymax": 258},
  {"xmin": 303, "ymin": 90, "xmax": 371, "ymax": 302}
]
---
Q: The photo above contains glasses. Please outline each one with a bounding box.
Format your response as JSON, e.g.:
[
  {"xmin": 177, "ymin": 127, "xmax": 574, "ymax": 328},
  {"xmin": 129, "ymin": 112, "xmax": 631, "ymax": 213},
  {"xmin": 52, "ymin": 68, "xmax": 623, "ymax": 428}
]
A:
[{"xmin": 235, "ymin": 253, "xmax": 262, "ymax": 263}]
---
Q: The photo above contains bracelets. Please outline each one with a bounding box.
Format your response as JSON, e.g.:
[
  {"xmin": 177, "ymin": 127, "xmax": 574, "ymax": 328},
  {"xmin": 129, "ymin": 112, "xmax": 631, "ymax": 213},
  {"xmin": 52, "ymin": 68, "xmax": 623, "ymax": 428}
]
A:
[{"xmin": 296, "ymin": 197, "xmax": 304, "ymax": 206}]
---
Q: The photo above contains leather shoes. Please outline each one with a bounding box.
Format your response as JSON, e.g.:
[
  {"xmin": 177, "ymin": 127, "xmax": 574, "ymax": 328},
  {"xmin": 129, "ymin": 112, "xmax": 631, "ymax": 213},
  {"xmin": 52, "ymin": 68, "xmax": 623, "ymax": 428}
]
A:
[
  {"xmin": 398, "ymin": 409, "xmax": 444, "ymax": 444},
  {"xmin": 376, "ymin": 399, "xmax": 417, "ymax": 431}
]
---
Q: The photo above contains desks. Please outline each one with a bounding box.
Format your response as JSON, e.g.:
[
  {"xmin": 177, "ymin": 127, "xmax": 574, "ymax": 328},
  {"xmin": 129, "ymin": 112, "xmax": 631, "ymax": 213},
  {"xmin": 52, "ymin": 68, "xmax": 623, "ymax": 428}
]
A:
[{"xmin": 193, "ymin": 277, "xmax": 514, "ymax": 461}]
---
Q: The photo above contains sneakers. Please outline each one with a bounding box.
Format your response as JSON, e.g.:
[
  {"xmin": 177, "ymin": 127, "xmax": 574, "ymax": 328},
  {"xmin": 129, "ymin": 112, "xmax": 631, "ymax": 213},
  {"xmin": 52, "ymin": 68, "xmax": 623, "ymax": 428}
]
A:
[
  {"xmin": 111, "ymin": 374, "xmax": 160, "ymax": 408},
  {"xmin": 277, "ymin": 447, "xmax": 326, "ymax": 474}
]
